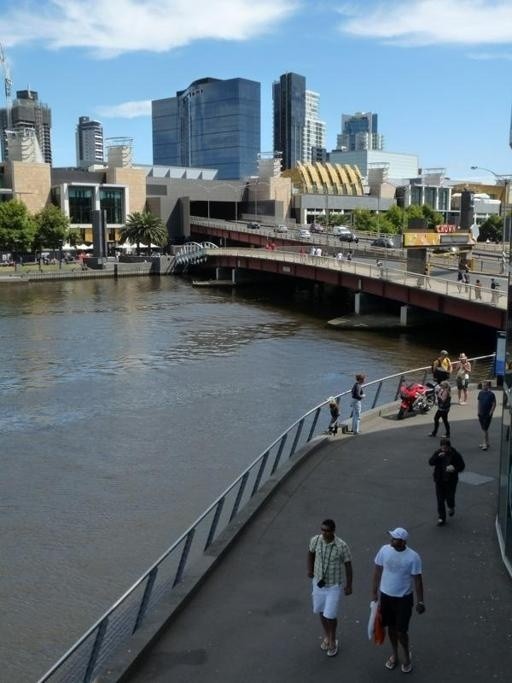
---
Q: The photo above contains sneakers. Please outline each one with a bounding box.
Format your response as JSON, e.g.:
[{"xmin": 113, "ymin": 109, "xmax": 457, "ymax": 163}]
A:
[
  {"xmin": 448, "ymin": 507, "xmax": 454, "ymax": 516},
  {"xmin": 427, "ymin": 433, "xmax": 435, "ymax": 437},
  {"xmin": 437, "ymin": 518, "xmax": 444, "ymax": 525},
  {"xmin": 440, "ymin": 436, "xmax": 450, "ymax": 439}
]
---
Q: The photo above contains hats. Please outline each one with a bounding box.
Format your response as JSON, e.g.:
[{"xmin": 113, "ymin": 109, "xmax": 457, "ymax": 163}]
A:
[
  {"xmin": 459, "ymin": 353, "xmax": 467, "ymax": 360},
  {"xmin": 386, "ymin": 527, "xmax": 408, "ymax": 541}
]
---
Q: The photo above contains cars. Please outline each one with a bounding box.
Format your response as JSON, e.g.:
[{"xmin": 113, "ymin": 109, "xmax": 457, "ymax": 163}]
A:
[
  {"xmin": 296, "ymin": 230, "xmax": 310, "ymax": 238},
  {"xmin": 310, "ymin": 223, "xmax": 323, "ymax": 233},
  {"xmin": 248, "ymin": 222, "xmax": 259, "ymax": 228},
  {"xmin": 372, "ymin": 238, "xmax": 394, "ymax": 247},
  {"xmin": 340, "ymin": 234, "xmax": 358, "ymax": 242},
  {"xmin": 272, "ymin": 224, "xmax": 287, "ymax": 233}
]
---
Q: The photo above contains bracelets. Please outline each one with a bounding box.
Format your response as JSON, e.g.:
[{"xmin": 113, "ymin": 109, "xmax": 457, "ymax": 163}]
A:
[{"xmin": 416, "ymin": 602, "xmax": 424, "ymax": 605}]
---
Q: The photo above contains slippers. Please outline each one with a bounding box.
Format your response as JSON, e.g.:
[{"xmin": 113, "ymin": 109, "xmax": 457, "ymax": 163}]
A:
[
  {"xmin": 321, "ymin": 639, "xmax": 338, "ymax": 656},
  {"xmin": 386, "ymin": 656, "xmax": 397, "ymax": 670},
  {"xmin": 478, "ymin": 443, "xmax": 489, "ymax": 450},
  {"xmin": 402, "ymin": 652, "xmax": 411, "ymax": 672}
]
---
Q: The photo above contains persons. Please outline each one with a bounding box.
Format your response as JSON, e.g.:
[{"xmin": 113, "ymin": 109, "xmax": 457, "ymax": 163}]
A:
[
  {"xmin": 433, "ymin": 350, "xmax": 453, "ymax": 384},
  {"xmin": 456, "ymin": 353, "xmax": 472, "ymax": 405},
  {"xmin": 477, "ymin": 381, "xmax": 496, "ymax": 450},
  {"xmin": 266, "ymin": 241, "xmax": 501, "ymax": 303},
  {"xmin": 427, "ymin": 381, "xmax": 451, "ymax": 438},
  {"xmin": 428, "ymin": 438, "xmax": 466, "ymax": 527},
  {"xmin": 351, "ymin": 374, "xmax": 367, "ymax": 434},
  {"xmin": 307, "ymin": 519, "xmax": 353, "ymax": 657},
  {"xmin": 372, "ymin": 527, "xmax": 426, "ymax": 673}
]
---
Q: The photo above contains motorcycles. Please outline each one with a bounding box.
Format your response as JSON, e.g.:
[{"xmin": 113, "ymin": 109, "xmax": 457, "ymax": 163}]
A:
[{"xmin": 397, "ymin": 380, "xmax": 439, "ymax": 420}]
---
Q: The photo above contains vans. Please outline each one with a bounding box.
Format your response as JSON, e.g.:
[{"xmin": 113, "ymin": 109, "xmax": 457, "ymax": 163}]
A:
[{"xmin": 333, "ymin": 226, "xmax": 352, "ymax": 236}]
